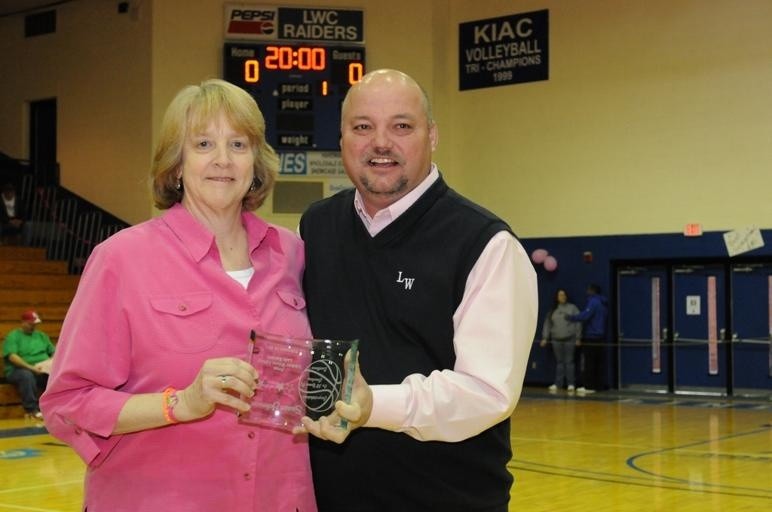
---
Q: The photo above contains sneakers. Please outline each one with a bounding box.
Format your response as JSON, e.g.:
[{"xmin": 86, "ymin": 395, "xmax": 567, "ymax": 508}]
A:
[
  {"xmin": 568, "ymin": 385, "xmax": 574, "ymax": 390},
  {"xmin": 549, "ymin": 384, "xmax": 557, "ymax": 390}
]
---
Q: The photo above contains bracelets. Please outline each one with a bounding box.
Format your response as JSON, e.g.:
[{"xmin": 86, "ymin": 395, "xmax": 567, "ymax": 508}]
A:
[{"xmin": 162, "ymin": 386, "xmax": 179, "ymax": 427}]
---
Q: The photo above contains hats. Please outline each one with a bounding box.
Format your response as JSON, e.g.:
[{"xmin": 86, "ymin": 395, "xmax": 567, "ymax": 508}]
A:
[{"xmin": 23, "ymin": 309, "xmax": 41, "ymax": 325}]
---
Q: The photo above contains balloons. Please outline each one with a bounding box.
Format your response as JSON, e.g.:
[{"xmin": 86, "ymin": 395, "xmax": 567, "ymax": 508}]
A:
[
  {"xmin": 543, "ymin": 256, "xmax": 560, "ymax": 272},
  {"xmin": 532, "ymin": 248, "xmax": 549, "ymax": 264}
]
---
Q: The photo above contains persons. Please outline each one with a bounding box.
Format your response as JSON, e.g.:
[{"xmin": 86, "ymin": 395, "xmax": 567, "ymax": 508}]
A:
[
  {"xmin": 38, "ymin": 77, "xmax": 319, "ymax": 511},
  {"xmin": 539, "ymin": 286, "xmax": 583, "ymax": 392},
  {"xmin": 548, "ymin": 391, "xmax": 585, "ymax": 397},
  {"xmin": 0, "ymin": 307, "xmax": 56, "ymax": 418},
  {"xmin": 296, "ymin": 63, "xmax": 543, "ymax": 511},
  {"xmin": 565, "ymin": 281, "xmax": 609, "ymax": 394},
  {"xmin": 0, "ymin": 181, "xmax": 29, "ymax": 246}
]
